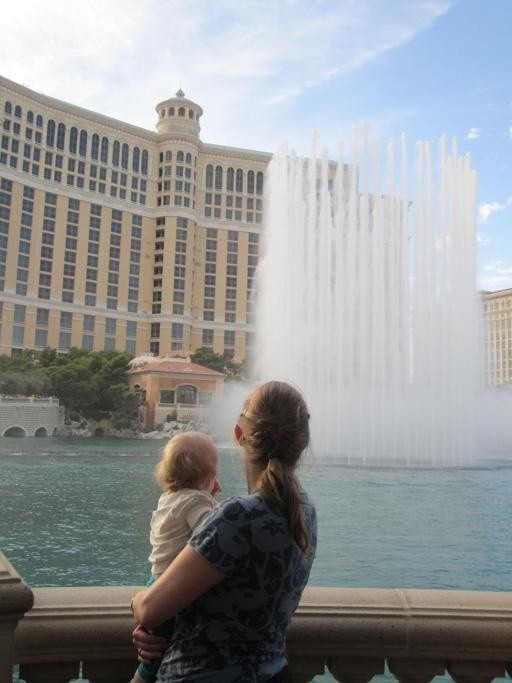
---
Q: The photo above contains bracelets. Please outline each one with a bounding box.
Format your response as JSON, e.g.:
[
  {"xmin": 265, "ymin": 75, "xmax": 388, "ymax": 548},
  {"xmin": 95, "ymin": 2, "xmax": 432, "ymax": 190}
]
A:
[{"xmin": 131, "ymin": 599, "xmax": 134, "ymax": 611}]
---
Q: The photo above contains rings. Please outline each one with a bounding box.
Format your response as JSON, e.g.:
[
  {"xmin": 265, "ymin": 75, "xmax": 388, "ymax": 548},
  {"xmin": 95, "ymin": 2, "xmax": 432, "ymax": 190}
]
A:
[{"xmin": 137, "ymin": 647, "xmax": 142, "ymax": 656}]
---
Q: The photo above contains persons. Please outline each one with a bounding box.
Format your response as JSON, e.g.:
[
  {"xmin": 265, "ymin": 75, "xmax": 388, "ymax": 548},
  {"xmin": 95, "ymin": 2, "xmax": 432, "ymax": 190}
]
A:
[
  {"xmin": 130, "ymin": 431, "xmax": 221, "ymax": 683},
  {"xmin": 132, "ymin": 382, "xmax": 317, "ymax": 682}
]
